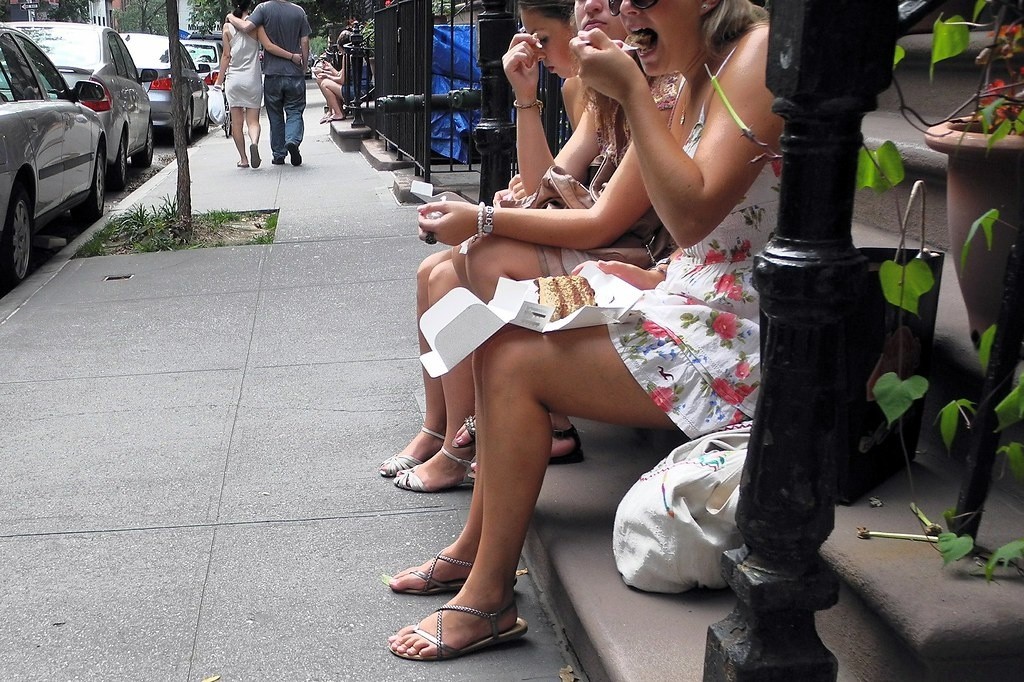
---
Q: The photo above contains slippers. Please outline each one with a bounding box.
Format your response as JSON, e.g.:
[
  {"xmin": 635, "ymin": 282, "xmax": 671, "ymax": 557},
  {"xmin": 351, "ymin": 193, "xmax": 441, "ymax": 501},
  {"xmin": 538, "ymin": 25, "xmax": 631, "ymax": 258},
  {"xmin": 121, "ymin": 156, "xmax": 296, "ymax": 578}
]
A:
[
  {"xmin": 238, "ymin": 161, "xmax": 249, "ymax": 167},
  {"xmin": 250, "ymin": 144, "xmax": 262, "ymax": 168},
  {"xmin": 326, "ymin": 116, "xmax": 346, "ymax": 123}
]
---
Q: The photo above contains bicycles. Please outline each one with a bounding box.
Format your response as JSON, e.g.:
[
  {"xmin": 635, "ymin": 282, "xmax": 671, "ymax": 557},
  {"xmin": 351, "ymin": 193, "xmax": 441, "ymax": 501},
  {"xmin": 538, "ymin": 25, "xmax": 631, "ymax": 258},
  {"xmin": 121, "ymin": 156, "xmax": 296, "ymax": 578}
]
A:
[{"xmin": 224, "ymin": 97, "xmax": 233, "ymax": 136}]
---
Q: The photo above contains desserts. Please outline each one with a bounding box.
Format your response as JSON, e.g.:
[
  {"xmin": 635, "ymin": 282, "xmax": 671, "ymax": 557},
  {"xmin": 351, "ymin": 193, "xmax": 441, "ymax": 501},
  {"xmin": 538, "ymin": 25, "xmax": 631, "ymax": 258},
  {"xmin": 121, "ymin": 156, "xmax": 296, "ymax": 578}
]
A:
[
  {"xmin": 533, "ymin": 274, "xmax": 598, "ymax": 323},
  {"xmin": 624, "ymin": 32, "xmax": 653, "ymax": 50}
]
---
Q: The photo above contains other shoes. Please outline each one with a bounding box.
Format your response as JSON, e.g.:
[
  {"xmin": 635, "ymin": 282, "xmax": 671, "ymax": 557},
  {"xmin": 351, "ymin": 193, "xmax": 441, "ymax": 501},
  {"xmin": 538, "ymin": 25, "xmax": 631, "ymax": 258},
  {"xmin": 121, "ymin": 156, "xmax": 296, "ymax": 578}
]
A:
[
  {"xmin": 319, "ymin": 112, "xmax": 333, "ymax": 124},
  {"xmin": 272, "ymin": 158, "xmax": 285, "ymax": 165},
  {"xmin": 285, "ymin": 142, "xmax": 302, "ymax": 166}
]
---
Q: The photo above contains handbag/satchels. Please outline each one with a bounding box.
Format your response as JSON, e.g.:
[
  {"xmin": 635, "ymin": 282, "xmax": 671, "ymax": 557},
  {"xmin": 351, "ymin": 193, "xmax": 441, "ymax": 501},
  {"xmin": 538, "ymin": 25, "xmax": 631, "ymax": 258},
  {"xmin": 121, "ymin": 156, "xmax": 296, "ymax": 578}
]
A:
[
  {"xmin": 836, "ymin": 179, "xmax": 947, "ymax": 504},
  {"xmin": 494, "ymin": 157, "xmax": 678, "ymax": 269},
  {"xmin": 614, "ymin": 421, "xmax": 750, "ymax": 594},
  {"xmin": 208, "ymin": 89, "xmax": 225, "ymax": 125}
]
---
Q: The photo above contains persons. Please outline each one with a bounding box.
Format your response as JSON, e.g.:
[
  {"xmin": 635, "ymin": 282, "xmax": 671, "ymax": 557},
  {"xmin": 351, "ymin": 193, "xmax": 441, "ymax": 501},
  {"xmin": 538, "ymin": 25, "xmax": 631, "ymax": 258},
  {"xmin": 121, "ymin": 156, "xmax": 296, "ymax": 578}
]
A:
[
  {"xmin": 378, "ymin": 0, "xmax": 785, "ymax": 662},
  {"xmin": 214, "ymin": 0, "xmax": 312, "ymax": 168},
  {"xmin": 311, "ymin": 30, "xmax": 373, "ymax": 125}
]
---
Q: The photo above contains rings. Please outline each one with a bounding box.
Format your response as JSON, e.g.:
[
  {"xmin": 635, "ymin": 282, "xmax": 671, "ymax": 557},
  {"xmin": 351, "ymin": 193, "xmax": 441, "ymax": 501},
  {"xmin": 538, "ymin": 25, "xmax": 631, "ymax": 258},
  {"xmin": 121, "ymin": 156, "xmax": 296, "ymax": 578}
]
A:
[{"xmin": 425, "ymin": 232, "xmax": 437, "ymax": 244}]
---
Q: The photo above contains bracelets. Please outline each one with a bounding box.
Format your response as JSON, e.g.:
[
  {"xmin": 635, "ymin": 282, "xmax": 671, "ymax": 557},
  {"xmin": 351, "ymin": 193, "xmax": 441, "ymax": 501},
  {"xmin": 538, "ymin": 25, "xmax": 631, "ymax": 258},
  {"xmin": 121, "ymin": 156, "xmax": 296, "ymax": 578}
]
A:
[
  {"xmin": 290, "ymin": 53, "xmax": 294, "ymax": 62},
  {"xmin": 647, "ymin": 267, "xmax": 667, "ymax": 281},
  {"xmin": 513, "ymin": 98, "xmax": 543, "ymax": 115},
  {"xmin": 322, "ymin": 71, "xmax": 324, "ymax": 73},
  {"xmin": 656, "ymin": 258, "xmax": 671, "ymax": 267},
  {"xmin": 471, "ymin": 202, "xmax": 494, "ymax": 243}
]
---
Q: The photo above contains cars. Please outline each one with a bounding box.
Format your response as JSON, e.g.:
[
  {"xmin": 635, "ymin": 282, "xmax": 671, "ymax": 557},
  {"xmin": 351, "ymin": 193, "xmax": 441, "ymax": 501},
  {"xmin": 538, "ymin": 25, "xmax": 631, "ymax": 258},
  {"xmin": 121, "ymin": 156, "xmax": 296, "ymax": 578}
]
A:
[
  {"xmin": 0, "ymin": 22, "xmax": 159, "ymax": 192},
  {"xmin": 179, "ymin": 40, "xmax": 228, "ymax": 89},
  {"xmin": 0, "ymin": 26, "xmax": 108, "ymax": 293},
  {"xmin": 119, "ymin": 33, "xmax": 210, "ymax": 149}
]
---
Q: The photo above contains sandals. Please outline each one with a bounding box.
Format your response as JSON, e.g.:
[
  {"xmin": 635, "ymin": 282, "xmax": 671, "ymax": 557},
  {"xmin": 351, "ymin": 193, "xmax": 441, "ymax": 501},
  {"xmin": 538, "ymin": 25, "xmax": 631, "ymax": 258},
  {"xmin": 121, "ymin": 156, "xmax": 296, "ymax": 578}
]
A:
[
  {"xmin": 469, "ymin": 424, "xmax": 584, "ymax": 477},
  {"xmin": 388, "ymin": 596, "xmax": 528, "ymax": 660},
  {"xmin": 451, "ymin": 415, "xmax": 476, "ymax": 450},
  {"xmin": 392, "ymin": 546, "xmax": 518, "ymax": 596},
  {"xmin": 380, "ymin": 427, "xmax": 445, "ymax": 477},
  {"xmin": 393, "ymin": 447, "xmax": 476, "ymax": 493}
]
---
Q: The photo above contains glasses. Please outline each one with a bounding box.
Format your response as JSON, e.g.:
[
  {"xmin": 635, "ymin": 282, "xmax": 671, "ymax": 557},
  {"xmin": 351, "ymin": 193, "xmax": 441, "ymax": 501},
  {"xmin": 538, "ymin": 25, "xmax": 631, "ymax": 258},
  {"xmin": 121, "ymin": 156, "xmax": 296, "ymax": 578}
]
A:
[{"xmin": 608, "ymin": 0, "xmax": 658, "ymax": 17}]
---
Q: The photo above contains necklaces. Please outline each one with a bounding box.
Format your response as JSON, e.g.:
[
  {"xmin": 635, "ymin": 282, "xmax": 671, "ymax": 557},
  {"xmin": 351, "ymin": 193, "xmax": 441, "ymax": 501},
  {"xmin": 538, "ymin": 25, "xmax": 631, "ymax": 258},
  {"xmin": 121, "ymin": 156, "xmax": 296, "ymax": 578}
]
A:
[{"xmin": 680, "ymin": 85, "xmax": 695, "ymax": 125}]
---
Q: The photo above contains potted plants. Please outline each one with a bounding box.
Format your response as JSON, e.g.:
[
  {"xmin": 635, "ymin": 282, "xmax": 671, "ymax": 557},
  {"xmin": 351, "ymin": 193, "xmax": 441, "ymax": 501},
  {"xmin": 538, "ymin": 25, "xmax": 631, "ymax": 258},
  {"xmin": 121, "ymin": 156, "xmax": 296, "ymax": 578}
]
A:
[{"xmin": 712, "ymin": 0, "xmax": 1024, "ymax": 580}]
